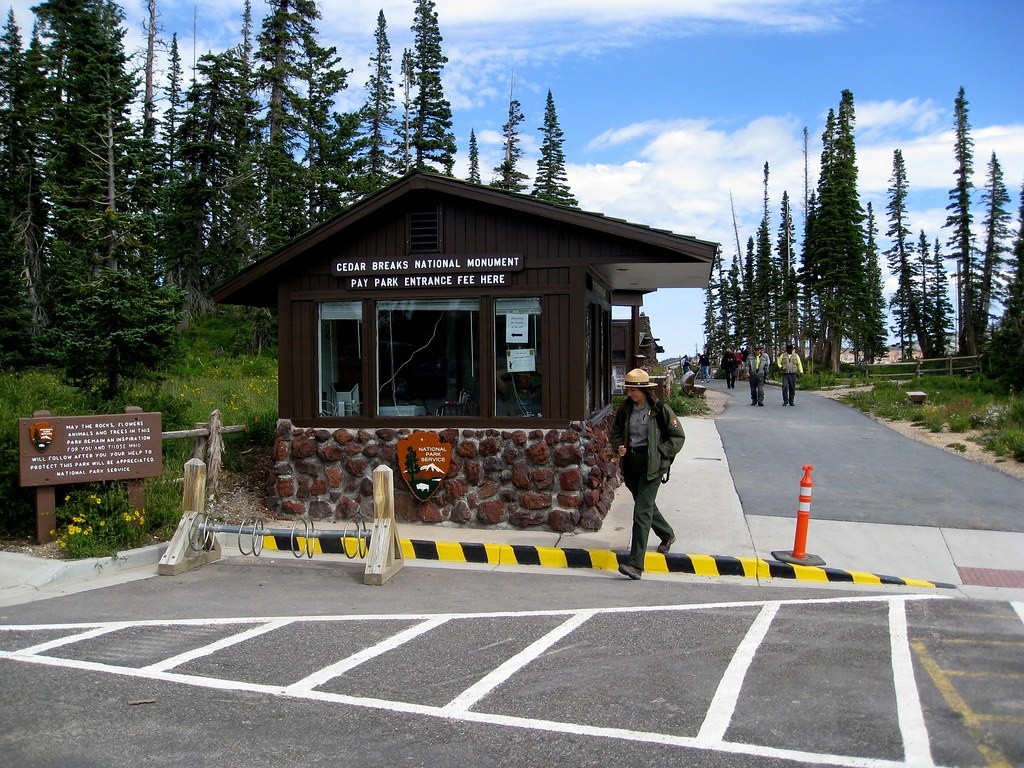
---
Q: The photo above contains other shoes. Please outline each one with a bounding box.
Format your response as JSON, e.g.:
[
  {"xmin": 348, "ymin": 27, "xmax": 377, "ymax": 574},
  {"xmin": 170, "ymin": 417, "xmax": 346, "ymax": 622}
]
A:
[
  {"xmin": 701, "ymin": 379, "xmax": 710, "ymax": 383},
  {"xmin": 726, "ymin": 384, "xmax": 735, "ymax": 389}
]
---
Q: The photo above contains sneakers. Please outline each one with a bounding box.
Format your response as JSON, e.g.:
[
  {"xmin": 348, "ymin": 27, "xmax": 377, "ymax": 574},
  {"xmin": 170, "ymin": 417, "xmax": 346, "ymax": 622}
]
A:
[
  {"xmin": 658, "ymin": 532, "xmax": 676, "ymax": 553},
  {"xmin": 751, "ymin": 399, "xmax": 764, "ymax": 407},
  {"xmin": 618, "ymin": 563, "xmax": 643, "ymax": 581},
  {"xmin": 781, "ymin": 400, "xmax": 796, "ymax": 407}
]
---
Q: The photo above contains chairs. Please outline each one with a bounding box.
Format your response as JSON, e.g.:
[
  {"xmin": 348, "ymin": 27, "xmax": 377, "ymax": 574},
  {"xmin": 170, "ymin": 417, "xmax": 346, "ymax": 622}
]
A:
[{"xmin": 435, "ymin": 402, "xmax": 467, "ymax": 416}]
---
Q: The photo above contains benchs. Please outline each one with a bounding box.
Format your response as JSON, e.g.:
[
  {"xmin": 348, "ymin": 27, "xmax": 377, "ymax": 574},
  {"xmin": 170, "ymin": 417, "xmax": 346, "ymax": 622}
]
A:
[
  {"xmin": 906, "ymin": 391, "xmax": 927, "ymax": 403},
  {"xmin": 680, "ymin": 370, "xmax": 707, "ymax": 397}
]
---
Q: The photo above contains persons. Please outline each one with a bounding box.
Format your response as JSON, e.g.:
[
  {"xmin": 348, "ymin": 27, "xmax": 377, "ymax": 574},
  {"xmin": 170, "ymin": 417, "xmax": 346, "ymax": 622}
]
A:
[
  {"xmin": 777, "ymin": 342, "xmax": 806, "ymax": 407},
  {"xmin": 744, "ymin": 346, "xmax": 768, "ymax": 407},
  {"xmin": 678, "ymin": 346, "xmax": 770, "ymax": 390},
  {"xmin": 608, "ymin": 368, "xmax": 685, "ymax": 580}
]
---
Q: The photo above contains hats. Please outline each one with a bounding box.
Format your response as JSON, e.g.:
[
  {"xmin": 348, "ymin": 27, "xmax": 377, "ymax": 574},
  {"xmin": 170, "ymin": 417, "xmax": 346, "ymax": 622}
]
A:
[{"xmin": 618, "ymin": 368, "xmax": 656, "ymax": 389}]
